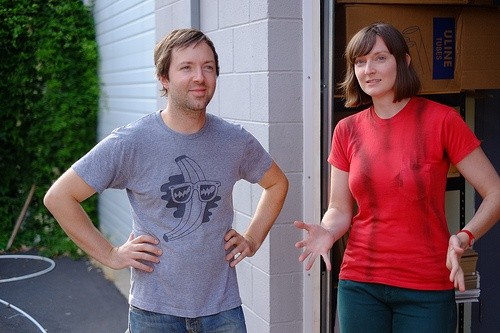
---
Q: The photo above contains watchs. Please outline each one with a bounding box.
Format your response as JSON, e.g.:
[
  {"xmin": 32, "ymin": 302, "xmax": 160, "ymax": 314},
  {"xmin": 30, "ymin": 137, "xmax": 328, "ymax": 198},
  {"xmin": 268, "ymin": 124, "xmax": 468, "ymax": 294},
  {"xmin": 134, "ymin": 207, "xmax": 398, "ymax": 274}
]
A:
[{"xmin": 455, "ymin": 230, "xmax": 475, "ymax": 248}]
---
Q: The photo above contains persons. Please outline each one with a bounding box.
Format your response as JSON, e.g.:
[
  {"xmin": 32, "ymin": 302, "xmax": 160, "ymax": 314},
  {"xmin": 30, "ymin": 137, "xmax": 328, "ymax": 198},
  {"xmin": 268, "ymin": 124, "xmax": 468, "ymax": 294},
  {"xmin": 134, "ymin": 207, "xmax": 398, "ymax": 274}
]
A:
[
  {"xmin": 294, "ymin": 22, "xmax": 500, "ymax": 333},
  {"xmin": 43, "ymin": 29, "xmax": 290, "ymax": 333}
]
